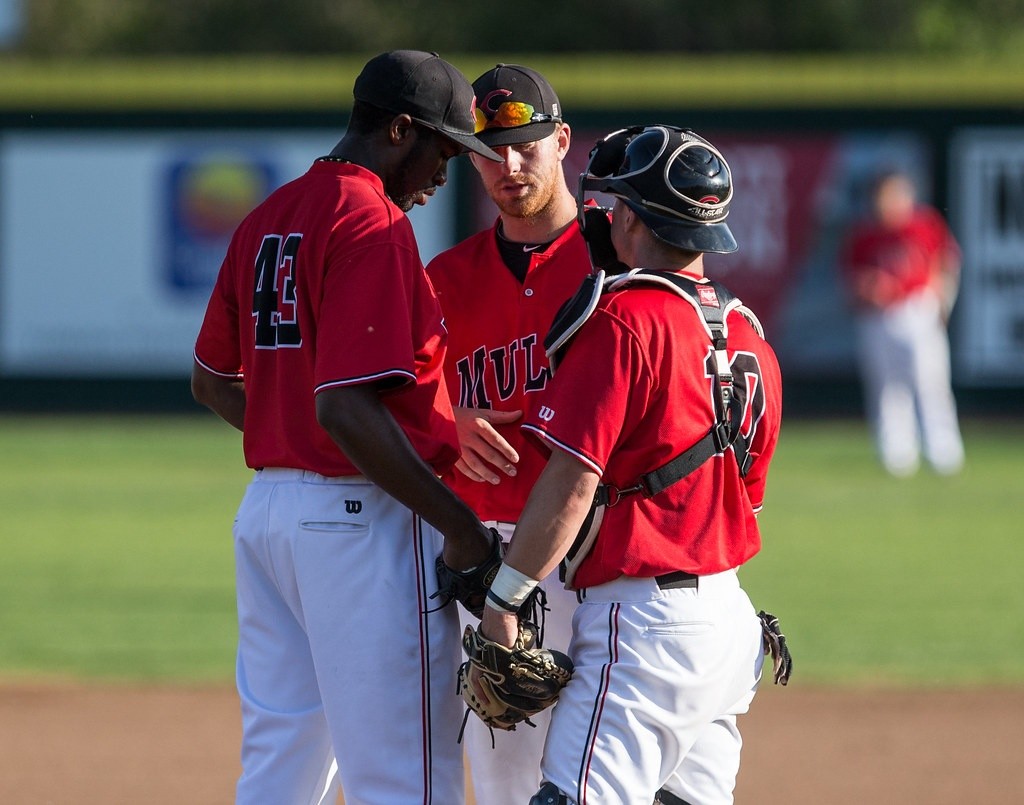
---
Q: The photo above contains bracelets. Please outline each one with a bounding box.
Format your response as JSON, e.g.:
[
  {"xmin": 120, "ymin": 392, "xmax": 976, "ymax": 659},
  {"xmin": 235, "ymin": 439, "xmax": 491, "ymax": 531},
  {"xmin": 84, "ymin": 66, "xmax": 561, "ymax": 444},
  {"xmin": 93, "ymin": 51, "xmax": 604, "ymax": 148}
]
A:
[
  {"xmin": 487, "ymin": 589, "xmax": 519, "ymax": 612},
  {"xmin": 485, "ymin": 562, "xmax": 540, "ymax": 612}
]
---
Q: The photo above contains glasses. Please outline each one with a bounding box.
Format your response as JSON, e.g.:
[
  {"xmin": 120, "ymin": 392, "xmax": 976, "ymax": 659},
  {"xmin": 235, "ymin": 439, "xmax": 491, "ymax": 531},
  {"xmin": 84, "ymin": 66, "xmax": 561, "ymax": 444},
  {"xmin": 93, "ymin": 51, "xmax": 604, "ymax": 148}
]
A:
[{"xmin": 470, "ymin": 101, "xmax": 563, "ymax": 132}]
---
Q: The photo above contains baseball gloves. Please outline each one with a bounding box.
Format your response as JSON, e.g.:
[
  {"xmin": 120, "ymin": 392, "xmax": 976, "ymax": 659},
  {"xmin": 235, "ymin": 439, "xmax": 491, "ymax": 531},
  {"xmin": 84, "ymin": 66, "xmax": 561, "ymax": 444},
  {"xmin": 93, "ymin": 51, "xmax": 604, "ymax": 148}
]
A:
[
  {"xmin": 455, "ymin": 617, "xmax": 574, "ymax": 750},
  {"xmin": 420, "ymin": 526, "xmax": 551, "ymax": 649}
]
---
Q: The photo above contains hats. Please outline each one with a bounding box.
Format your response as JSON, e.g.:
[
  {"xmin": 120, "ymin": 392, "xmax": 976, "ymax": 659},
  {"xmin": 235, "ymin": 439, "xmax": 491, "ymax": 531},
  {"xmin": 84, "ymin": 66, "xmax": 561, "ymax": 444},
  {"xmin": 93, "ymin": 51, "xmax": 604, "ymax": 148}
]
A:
[
  {"xmin": 470, "ymin": 61, "xmax": 563, "ymax": 150},
  {"xmin": 352, "ymin": 45, "xmax": 509, "ymax": 169}
]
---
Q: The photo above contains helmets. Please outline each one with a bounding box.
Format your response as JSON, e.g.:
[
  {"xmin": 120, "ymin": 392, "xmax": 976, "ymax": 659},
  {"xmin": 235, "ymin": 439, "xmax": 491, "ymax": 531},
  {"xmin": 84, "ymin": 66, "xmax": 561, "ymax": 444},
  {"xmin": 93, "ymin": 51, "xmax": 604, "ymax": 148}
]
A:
[{"xmin": 577, "ymin": 122, "xmax": 739, "ymax": 254}]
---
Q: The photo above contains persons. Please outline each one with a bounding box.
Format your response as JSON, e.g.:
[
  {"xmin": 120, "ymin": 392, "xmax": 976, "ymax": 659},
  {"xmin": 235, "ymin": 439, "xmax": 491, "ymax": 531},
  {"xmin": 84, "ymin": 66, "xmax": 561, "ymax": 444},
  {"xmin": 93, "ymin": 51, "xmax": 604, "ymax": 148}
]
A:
[
  {"xmin": 456, "ymin": 125, "xmax": 781, "ymax": 805},
  {"xmin": 192, "ymin": 49, "xmax": 536, "ymax": 805},
  {"xmin": 425, "ymin": 65, "xmax": 611, "ymax": 804},
  {"xmin": 842, "ymin": 173, "xmax": 965, "ymax": 476}
]
